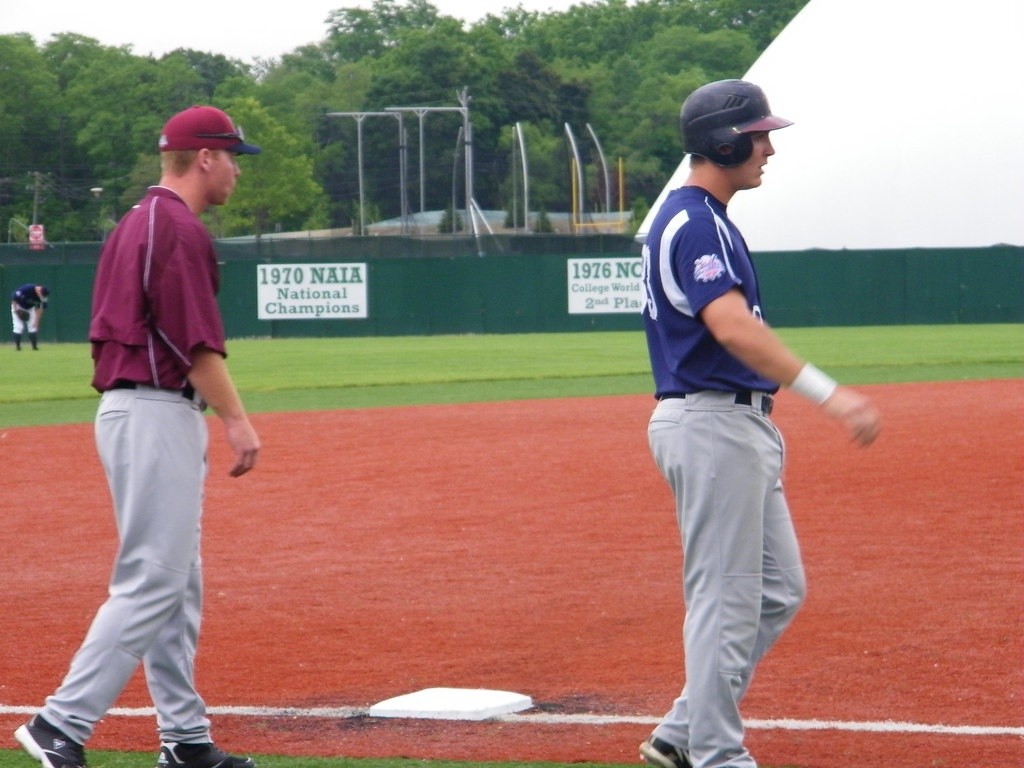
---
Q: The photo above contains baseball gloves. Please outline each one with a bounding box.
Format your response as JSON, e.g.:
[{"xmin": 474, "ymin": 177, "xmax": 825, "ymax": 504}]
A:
[{"xmin": 15, "ymin": 309, "xmax": 30, "ymax": 321}]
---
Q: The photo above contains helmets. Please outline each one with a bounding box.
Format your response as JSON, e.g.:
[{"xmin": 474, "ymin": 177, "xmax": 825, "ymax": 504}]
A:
[{"xmin": 681, "ymin": 79, "xmax": 792, "ymax": 168}]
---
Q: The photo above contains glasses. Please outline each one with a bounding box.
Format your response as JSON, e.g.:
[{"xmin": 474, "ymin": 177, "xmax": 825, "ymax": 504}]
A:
[{"xmin": 195, "ymin": 124, "xmax": 246, "ymax": 143}]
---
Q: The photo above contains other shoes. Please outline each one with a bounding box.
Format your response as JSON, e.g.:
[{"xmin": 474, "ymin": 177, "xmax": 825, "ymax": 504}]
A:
[
  {"xmin": 32, "ymin": 347, "xmax": 38, "ymax": 351},
  {"xmin": 15, "ymin": 347, "xmax": 21, "ymax": 351}
]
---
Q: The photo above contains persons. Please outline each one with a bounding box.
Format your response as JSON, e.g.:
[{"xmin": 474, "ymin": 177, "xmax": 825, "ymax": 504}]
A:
[
  {"xmin": 639, "ymin": 80, "xmax": 883, "ymax": 768},
  {"xmin": 14, "ymin": 106, "xmax": 262, "ymax": 768},
  {"xmin": 11, "ymin": 283, "xmax": 49, "ymax": 351}
]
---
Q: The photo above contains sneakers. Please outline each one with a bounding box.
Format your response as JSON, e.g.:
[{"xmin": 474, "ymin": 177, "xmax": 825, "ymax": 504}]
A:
[
  {"xmin": 154, "ymin": 740, "xmax": 256, "ymax": 768},
  {"xmin": 639, "ymin": 734, "xmax": 692, "ymax": 768},
  {"xmin": 12, "ymin": 714, "xmax": 88, "ymax": 768}
]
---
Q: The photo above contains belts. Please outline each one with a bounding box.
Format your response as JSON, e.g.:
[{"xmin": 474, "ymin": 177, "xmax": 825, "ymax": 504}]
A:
[
  {"xmin": 660, "ymin": 390, "xmax": 774, "ymax": 414},
  {"xmin": 116, "ymin": 379, "xmax": 209, "ymax": 412}
]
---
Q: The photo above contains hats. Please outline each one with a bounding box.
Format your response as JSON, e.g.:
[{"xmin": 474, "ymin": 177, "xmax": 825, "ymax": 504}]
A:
[
  {"xmin": 40, "ymin": 286, "xmax": 49, "ymax": 302},
  {"xmin": 160, "ymin": 104, "xmax": 262, "ymax": 155}
]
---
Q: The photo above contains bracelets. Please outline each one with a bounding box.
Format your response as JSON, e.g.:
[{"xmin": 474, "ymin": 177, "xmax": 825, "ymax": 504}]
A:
[{"xmin": 787, "ymin": 362, "xmax": 838, "ymax": 407}]
